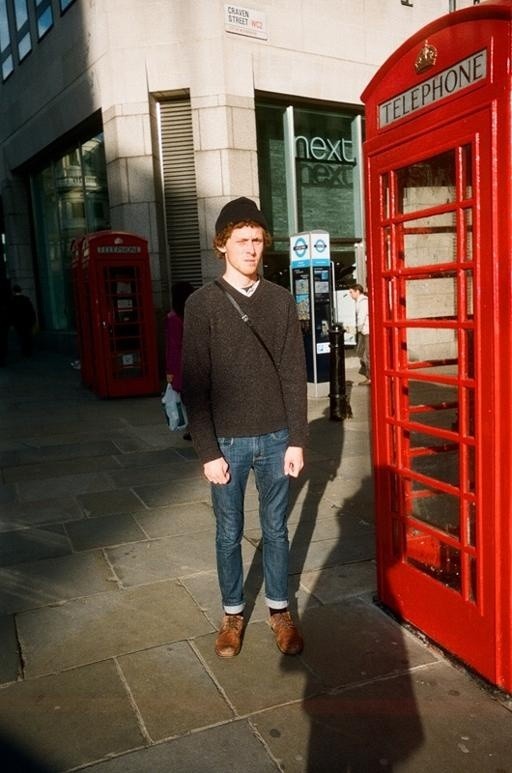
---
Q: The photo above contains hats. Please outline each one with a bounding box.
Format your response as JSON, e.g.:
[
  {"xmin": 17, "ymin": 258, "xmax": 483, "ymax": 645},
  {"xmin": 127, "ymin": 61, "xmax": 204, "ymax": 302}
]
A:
[{"xmin": 216, "ymin": 197, "xmax": 266, "ymax": 235}]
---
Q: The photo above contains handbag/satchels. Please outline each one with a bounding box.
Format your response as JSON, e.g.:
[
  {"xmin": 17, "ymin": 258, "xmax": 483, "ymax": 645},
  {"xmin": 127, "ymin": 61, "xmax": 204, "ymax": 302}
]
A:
[{"xmin": 162, "ymin": 383, "xmax": 188, "ymax": 430}]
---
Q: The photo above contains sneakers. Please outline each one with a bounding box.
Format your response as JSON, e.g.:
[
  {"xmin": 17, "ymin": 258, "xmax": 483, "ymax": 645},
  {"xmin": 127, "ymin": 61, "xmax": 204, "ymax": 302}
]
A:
[
  {"xmin": 216, "ymin": 613, "xmax": 244, "ymax": 657},
  {"xmin": 268, "ymin": 611, "xmax": 303, "ymax": 655}
]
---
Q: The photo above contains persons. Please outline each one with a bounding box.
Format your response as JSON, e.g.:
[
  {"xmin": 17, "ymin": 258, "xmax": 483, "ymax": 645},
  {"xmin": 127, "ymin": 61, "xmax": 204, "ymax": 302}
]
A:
[
  {"xmin": 349, "ymin": 284, "xmax": 372, "ymax": 385},
  {"xmin": 8, "ymin": 284, "xmax": 36, "ymax": 354},
  {"xmin": 163, "ymin": 281, "xmax": 195, "ymax": 441},
  {"xmin": 181, "ymin": 196, "xmax": 310, "ymax": 658}
]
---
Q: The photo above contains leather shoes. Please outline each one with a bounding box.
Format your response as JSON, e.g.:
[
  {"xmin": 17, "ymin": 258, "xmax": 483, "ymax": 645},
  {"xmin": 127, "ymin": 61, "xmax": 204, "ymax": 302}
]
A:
[{"xmin": 359, "ymin": 379, "xmax": 371, "ymax": 385}]
